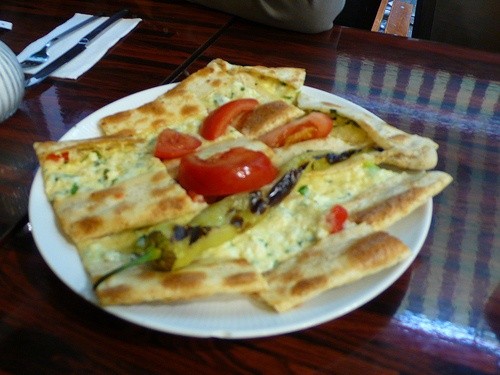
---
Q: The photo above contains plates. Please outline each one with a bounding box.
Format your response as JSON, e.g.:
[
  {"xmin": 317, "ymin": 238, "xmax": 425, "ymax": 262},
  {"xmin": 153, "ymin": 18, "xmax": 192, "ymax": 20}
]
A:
[{"xmin": 27, "ymin": 78, "xmax": 433, "ymax": 338}]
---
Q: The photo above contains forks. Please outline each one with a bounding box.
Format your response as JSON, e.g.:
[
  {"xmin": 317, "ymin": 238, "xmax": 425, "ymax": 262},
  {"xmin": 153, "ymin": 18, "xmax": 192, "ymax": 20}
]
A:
[{"xmin": 18, "ymin": 12, "xmax": 102, "ymax": 67}]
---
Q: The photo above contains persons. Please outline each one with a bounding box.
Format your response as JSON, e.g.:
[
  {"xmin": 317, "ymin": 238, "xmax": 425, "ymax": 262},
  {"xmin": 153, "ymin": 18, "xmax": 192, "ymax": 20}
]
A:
[{"xmin": 185, "ymin": 1, "xmax": 346, "ymax": 33}]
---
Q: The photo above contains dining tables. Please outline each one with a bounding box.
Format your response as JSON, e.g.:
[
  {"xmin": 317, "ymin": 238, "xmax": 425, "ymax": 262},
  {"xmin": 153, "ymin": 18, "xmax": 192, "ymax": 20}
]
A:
[{"xmin": 1, "ymin": 0, "xmax": 499, "ymax": 375}]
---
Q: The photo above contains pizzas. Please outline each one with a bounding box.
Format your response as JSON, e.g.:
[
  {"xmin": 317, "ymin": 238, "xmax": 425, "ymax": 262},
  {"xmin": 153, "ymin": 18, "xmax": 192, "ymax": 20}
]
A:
[{"xmin": 33, "ymin": 58, "xmax": 453, "ymax": 313}]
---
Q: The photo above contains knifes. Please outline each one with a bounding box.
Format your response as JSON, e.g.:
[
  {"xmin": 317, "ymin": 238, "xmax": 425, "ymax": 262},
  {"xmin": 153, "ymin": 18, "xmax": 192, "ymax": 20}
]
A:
[{"xmin": 26, "ymin": 10, "xmax": 127, "ymax": 88}]
